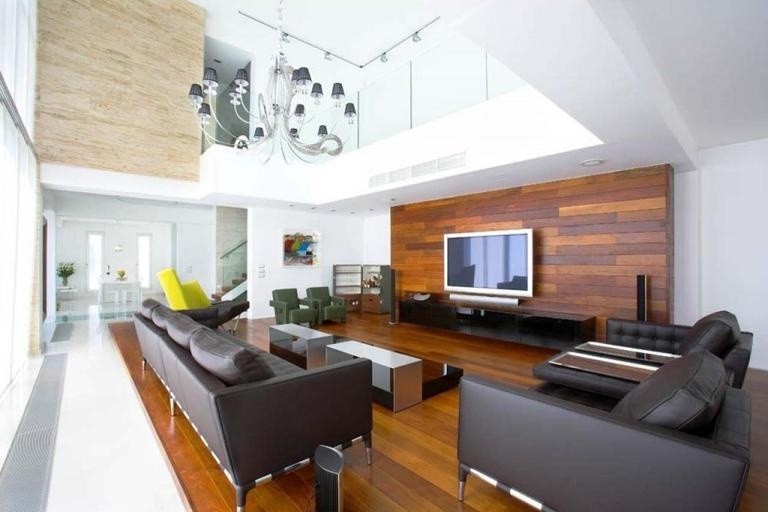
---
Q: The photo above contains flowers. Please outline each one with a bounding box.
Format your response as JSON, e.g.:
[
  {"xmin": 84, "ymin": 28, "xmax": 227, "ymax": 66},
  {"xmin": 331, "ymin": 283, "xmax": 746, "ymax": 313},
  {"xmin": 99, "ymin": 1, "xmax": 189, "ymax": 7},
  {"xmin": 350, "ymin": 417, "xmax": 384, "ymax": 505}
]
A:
[{"xmin": 55, "ymin": 261, "xmax": 74, "ymax": 278}]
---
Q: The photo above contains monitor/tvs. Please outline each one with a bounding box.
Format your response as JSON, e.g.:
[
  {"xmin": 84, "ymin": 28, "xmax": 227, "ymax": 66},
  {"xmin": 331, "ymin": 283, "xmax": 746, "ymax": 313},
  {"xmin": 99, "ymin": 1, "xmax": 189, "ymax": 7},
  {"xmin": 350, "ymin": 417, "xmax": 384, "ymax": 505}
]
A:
[{"xmin": 443, "ymin": 229, "xmax": 533, "ymax": 305}]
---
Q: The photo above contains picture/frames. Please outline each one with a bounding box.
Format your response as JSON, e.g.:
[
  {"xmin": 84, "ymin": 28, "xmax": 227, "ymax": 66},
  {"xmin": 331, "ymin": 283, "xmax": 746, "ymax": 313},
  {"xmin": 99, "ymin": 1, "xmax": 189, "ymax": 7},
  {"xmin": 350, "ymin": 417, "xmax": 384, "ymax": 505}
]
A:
[{"xmin": 282, "ymin": 232, "xmax": 319, "ymax": 268}]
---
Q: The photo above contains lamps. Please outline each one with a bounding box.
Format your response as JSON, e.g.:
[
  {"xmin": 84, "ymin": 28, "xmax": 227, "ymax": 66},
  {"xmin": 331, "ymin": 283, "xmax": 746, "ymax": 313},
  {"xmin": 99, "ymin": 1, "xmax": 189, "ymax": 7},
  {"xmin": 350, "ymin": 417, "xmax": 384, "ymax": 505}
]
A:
[
  {"xmin": 380, "ymin": 52, "xmax": 387, "ymax": 62},
  {"xmin": 185, "ymin": 1, "xmax": 359, "ymax": 167},
  {"xmin": 323, "ymin": 50, "xmax": 334, "ymax": 62},
  {"xmin": 278, "ymin": 31, "xmax": 290, "ymax": 46},
  {"xmin": 413, "ymin": 29, "xmax": 419, "ymax": 42}
]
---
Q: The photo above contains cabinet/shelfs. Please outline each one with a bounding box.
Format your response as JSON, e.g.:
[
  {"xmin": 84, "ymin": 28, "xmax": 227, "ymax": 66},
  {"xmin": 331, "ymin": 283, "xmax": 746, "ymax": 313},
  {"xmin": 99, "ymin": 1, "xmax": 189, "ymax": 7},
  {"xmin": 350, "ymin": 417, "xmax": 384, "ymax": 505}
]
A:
[
  {"xmin": 362, "ymin": 264, "xmax": 393, "ymax": 314},
  {"xmin": 332, "ymin": 263, "xmax": 362, "ymax": 313}
]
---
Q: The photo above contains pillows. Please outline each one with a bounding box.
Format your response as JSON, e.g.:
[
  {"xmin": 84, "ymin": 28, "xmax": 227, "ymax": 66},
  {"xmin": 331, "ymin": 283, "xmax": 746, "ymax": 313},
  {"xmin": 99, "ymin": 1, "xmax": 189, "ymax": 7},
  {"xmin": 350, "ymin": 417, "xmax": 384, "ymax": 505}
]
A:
[
  {"xmin": 134, "ymin": 298, "xmax": 163, "ymax": 321},
  {"xmin": 151, "ymin": 304, "xmax": 196, "ymax": 331},
  {"xmin": 163, "ymin": 309, "xmax": 213, "ymax": 348},
  {"xmin": 678, "ymin": 309, "xmax": 744, "ymax": 356},
  {"xmin": 605, "ymin": 348, "xmax": 729, "ymax": 433},
  {"xmin": 189, "ymin": 323, "xmax": 272, "ymax": 383}
]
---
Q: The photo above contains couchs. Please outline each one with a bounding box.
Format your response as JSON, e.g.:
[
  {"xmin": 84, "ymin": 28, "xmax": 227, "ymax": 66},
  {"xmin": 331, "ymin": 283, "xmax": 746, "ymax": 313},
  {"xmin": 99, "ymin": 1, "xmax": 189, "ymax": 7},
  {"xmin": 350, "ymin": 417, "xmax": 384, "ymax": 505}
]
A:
[
  {"xmin": 306, "ymin": 287, "xmax": 345, "ymax": 326},
  {"xmin": 131, "ymin": 313, "xmax": 373, "ymax": 511},
  {"xmin": 458, "ymin": 315, "xmax": 753, "ymax": 512},
  {"xmin": 270, "ymin": 288, "xmax": 319, "ymax": 329},
  {"xmin": 156, "ymin": 267, "xmax": 252, "ymax": 337}
]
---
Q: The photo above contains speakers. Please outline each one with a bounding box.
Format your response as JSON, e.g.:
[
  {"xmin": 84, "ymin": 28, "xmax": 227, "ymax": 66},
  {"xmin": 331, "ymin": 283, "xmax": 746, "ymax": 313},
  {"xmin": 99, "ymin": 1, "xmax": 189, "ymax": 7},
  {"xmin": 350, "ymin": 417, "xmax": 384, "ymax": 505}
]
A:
[
  {"xmin": 389, "ymin": 269, "xmax": 397, "ymax": 326},
  {"xmin": 636, "ymin": 273, "xmax": 647, "ymax": 360}
]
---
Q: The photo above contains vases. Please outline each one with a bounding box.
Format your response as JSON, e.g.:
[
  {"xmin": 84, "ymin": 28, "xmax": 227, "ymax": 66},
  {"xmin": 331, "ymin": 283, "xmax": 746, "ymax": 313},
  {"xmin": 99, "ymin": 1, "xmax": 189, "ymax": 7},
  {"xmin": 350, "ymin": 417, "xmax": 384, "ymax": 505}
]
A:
[{"xmin": 62, "ymin": 278, "xmax": 70, "ymax": 287}]
